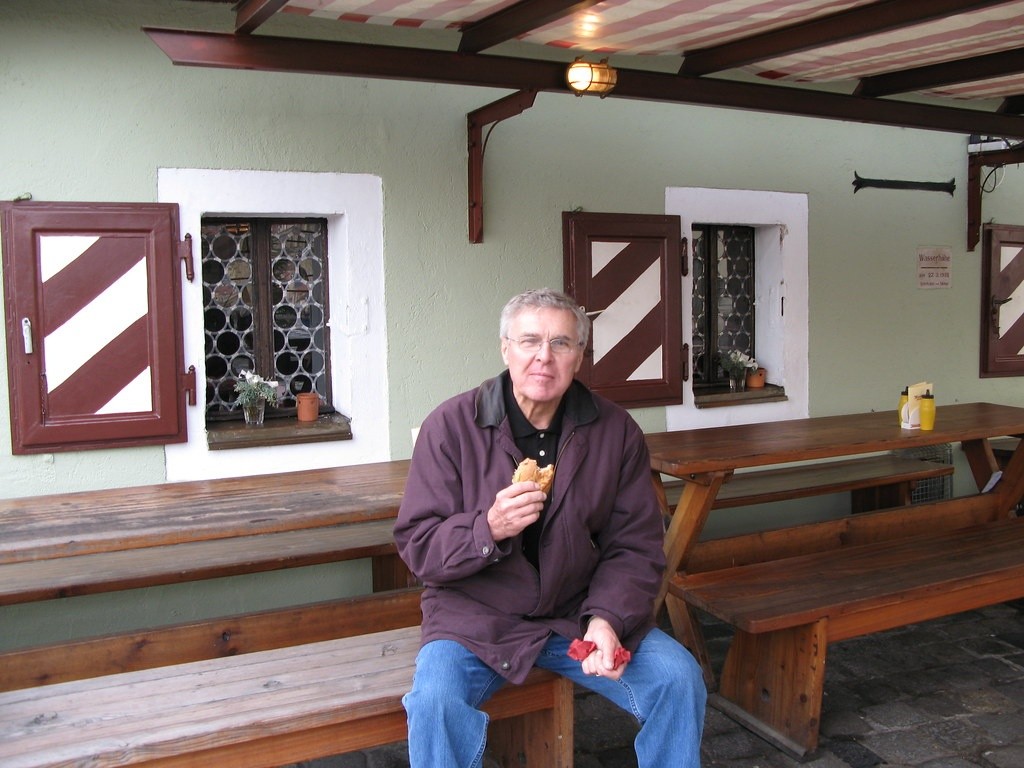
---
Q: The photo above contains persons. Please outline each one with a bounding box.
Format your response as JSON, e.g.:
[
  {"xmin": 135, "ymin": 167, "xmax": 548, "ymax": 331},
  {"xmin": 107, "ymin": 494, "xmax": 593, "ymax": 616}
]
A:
[{"xmin": 393, "ymin": 290, "xmax": 707, "ymax": 768}]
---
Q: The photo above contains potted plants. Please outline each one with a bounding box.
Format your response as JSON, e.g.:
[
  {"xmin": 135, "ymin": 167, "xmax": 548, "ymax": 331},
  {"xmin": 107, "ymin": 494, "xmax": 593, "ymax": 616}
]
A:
[{"xmin": 718, "ymin": 349, "xmax": 757, "ymax": 379}]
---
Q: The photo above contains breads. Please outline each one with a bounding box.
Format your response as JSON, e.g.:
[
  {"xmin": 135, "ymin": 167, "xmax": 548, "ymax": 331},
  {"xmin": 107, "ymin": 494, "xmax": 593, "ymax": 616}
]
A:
[{"xmin": 511, "ymin": 458, "xmax": 554, "ymax": 496}]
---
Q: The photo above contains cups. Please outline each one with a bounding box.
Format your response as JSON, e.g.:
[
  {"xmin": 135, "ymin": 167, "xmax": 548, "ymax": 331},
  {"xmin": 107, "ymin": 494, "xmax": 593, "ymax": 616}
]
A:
[
  {"xmin": 746, "ymin": 368, "xmax": 765, "ymax": 387},
  {"xmin": 297, "ymin": 393, "xmax": 318, "ymax": 422}
]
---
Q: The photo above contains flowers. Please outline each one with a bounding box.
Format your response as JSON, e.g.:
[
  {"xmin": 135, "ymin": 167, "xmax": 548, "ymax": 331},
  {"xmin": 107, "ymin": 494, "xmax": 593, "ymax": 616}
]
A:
[{"xmin": 233, "ymin": 370, "xmax": 280, "ymax": 416}]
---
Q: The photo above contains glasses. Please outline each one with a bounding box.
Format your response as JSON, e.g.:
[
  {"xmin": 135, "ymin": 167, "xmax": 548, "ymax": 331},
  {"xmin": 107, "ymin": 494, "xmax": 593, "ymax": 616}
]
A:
[{"xmin": 507, "ymin": 337, "xmax": 583, "ymax": 354}]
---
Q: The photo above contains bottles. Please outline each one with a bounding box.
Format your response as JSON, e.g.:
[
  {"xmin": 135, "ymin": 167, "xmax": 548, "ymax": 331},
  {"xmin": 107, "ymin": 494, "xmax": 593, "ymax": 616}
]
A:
[
  {"xmin": 897, "ymin": 386, "xmax": 908, "ymax": 426},
  {"xmin": 920, "ymin": 389, "xmax": 935, "ymax": 431}
]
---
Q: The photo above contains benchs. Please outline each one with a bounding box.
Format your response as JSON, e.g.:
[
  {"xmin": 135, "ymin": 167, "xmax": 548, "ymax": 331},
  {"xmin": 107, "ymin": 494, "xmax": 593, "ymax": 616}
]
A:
[
  {"xmin": 0, "ymin": 516, "xmax": 421, "ymax": 596},
  {"xmin": 667, "ymin": 514, "xmax": 1024, "ymax": 762},
  {"xmin": 661, "ymin": 455, "xmax": 955, "ymax": 513},
  {"xmin": 0, "ymin": 622, "xmax": 575, "ymax": 768}
]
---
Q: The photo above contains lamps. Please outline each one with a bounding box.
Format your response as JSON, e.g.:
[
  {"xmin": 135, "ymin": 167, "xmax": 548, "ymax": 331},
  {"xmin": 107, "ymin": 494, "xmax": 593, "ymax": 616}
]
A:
[{"xmin": 565, "ymin": 55, "xmax": 618, "ymax": 99}]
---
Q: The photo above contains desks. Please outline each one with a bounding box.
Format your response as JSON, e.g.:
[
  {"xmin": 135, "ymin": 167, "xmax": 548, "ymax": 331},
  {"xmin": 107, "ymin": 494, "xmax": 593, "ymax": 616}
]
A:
[
  {"xmin": 641, "ymin": 402, "xmax": 1024, "ymax": 696},
  {"xmin": 0, "ymin": 458, "xmax": 424, "ymax": 693}
]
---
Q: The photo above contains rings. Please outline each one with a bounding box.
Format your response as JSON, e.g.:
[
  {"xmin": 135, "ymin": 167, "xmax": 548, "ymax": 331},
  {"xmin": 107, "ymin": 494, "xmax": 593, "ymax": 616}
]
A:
[{"xmin": 596, "ymin": 674, "xmax": 600, "ymax": 676}]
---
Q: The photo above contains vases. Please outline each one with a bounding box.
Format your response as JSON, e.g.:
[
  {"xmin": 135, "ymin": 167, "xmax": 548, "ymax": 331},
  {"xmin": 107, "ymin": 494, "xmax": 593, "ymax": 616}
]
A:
[
  {"xmin": 747, "ymin": 368, "xmax": 767, "ymax": 387},
  {"xmin": 730, "ymin": 376, "xmax": 746, "ymax": 392},
  {"xmin": 241, "ymin": 396, "xmax": 266, "ymax": 425}
]
